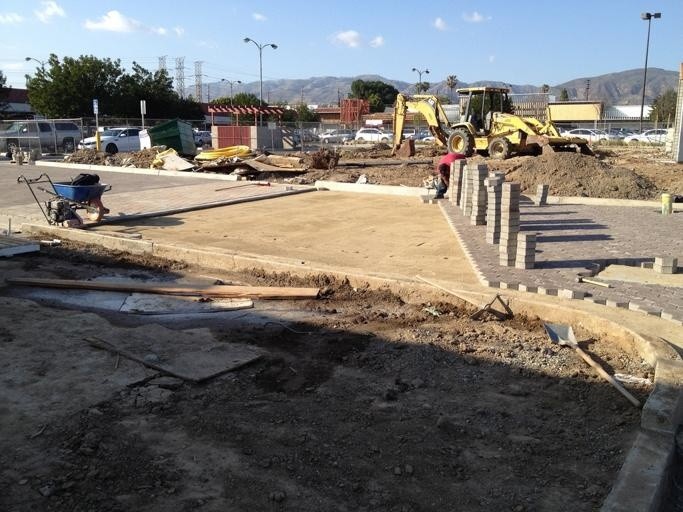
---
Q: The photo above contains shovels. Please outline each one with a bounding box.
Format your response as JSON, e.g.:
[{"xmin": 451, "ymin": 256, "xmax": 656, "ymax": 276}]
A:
[{"xmin": 543, "ymin": 323, "xmax": 641, "ymax": 407}]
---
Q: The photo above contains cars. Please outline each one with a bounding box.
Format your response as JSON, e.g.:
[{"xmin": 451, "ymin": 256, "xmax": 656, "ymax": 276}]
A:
[
  {"xmin": 292, "ymin": 125, "xmax": 431, "ymax": 143},
  {"xmin": 77, "ymin": 127, "xmax": 142, "ymax": 155},
  {"xmin": 562, "ymin": 128, "xmax": 673, "ymax": 143},
  {"xmin": 194, "ymin": 130, "xmax": 210, "ymax": 144}
]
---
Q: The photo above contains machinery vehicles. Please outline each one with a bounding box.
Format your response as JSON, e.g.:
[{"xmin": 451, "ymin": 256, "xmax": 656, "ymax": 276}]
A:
[{"xmin": 391, "ymin": 86, "xmax": 596, "ymax": 160}]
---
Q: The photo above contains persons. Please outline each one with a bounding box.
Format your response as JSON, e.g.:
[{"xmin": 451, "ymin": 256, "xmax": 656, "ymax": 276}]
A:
[{"xmin": 433, "ymin": 153, "xmax": 465, "ymax": 199}]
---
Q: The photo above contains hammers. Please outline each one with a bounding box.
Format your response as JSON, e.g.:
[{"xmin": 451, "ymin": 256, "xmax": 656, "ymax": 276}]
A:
[
  {"xmin": 576, "ymin": 275, "xmax": 612, "ymax": 288},
  {"xmin": 414, "ymin": 273, "xmax": 498, "ymax": 320}
]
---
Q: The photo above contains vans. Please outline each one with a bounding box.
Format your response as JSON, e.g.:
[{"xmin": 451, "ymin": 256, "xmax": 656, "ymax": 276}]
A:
[{"xmin": 0, "ymin": 118, "xmax": 82, "ymax": 151}]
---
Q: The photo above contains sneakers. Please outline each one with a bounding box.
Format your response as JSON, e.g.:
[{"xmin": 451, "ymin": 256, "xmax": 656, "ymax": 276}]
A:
[{"xmin": 434, "ymin": 195, "xmax": 444, "ymax": 199}]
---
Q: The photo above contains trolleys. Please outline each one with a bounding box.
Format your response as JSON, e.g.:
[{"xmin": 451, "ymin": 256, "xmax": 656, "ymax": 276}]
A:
[{"xmin": 17, "ymin": 174, "xmax": 112, "ymax": 228}]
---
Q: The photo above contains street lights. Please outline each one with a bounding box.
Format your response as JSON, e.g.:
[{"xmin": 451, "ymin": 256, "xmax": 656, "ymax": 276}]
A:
[
  {"xmin": 412, "ymin": 66, "xmax": 430, "ymax": 95},
  {"xmin": 25, "ymin": 57, "xmax": 45, "ymax": 67},
  {"xmin": 220, "ymin": 37, "xmax": 278, "ymax": 126},
  {"xmin": 640, "ymin": 11, "xmax": 661, "ymax": 134}
]
